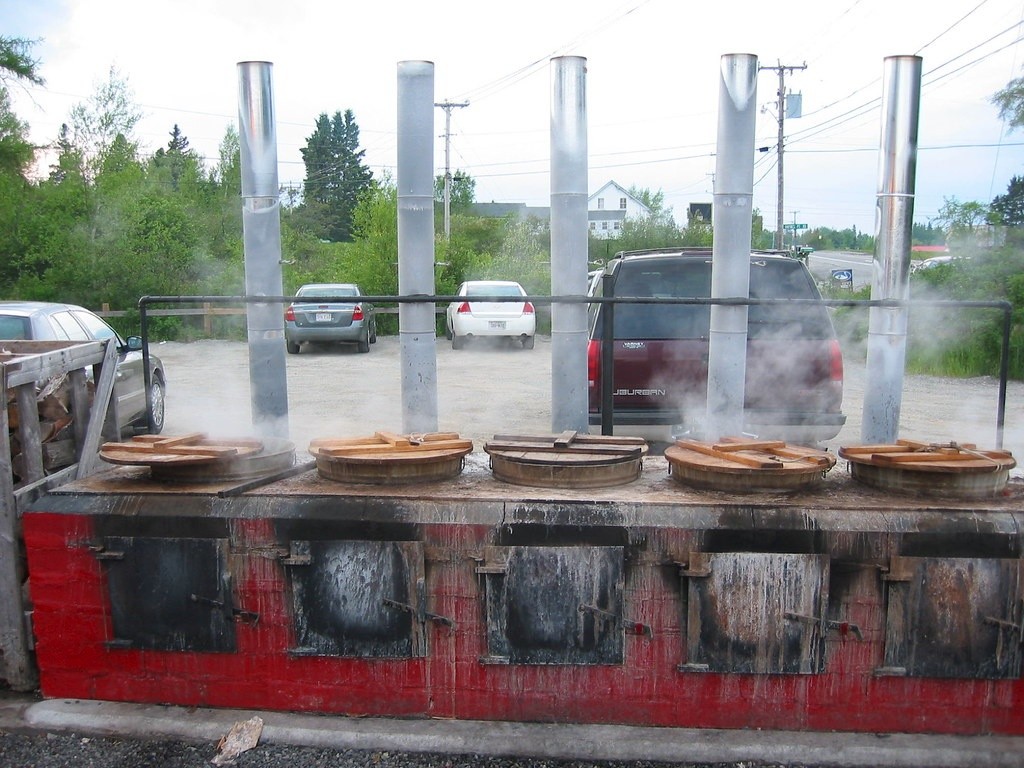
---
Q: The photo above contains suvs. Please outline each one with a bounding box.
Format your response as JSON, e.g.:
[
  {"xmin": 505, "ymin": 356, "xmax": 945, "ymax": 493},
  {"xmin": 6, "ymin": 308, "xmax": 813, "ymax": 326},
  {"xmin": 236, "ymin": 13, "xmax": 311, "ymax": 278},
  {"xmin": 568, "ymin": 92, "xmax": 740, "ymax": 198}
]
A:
[{"xmin": 586, "ymin": 245, "xmax": 848, "ymax": 442}]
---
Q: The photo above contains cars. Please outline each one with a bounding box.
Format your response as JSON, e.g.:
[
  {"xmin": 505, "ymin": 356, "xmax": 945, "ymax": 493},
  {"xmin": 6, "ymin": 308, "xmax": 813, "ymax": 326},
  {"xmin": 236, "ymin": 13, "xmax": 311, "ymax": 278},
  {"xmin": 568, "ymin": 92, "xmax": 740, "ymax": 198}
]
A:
[
  {"xmin": 0, "ymin": 299, "xmax": 167, "ymax": 436},
  {"xmin": 910, "ymin": 256, "xmax": 971, "ymax": 276},
  {"xmin": 446, "ymin": 280, "xmax": 536, "ymax": 350},
  {"xmin": 284, "ymin": 284, "xmax": 377, "ymax": 354}
]
